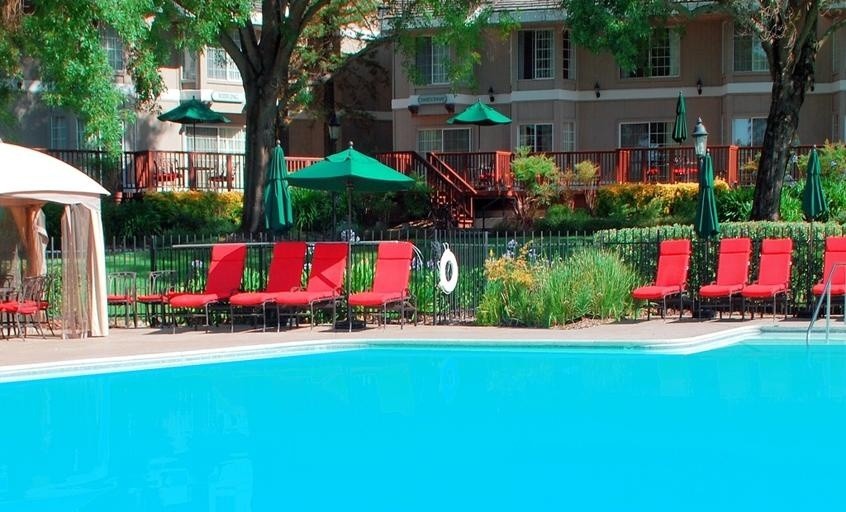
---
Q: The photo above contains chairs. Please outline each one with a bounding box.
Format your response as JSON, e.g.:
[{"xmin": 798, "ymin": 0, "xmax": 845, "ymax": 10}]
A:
[
  {"xmin": 141, "ymin": 268, "xmax": 179, "ymax": 331},
  {"xmin": 699, "ymin": 238, "xmax": 752, "ymax": 321},
  {"xmin": 230, "ymin": 243, "xmax": 307, "ymax": 332},
  {"xmin": 633, "ymin": 239, "xmax": 691, "ymax": 322},
  {"xmin": 25, "ymin": 275, "xmax": 55, "ymax": 337},
  {"xmin": 0, "ymin": 275, "xmax": 13, "ymax": 337},
  {"xmin": 107, "ymin": 272, "xmax": 138, "ymax": 329},
  {"xmin": 811, "ymin": 236, "xmax": 845, "ymax": 321},
  {"xmin": 742, "ymin": 238, "xmax": 794, "ymax": 321},
  {"xmin": 168, "ymin": 244, "xmax": 246, "ymax": 333},
  {"xmin": 348, "ymin": 241, "xmax": 413, "ymax": 330},
  {"xmin": 273, "ymin": 243, "xmax": 350, "ymax": 330},
  {"xmin": 1, "ymin": 273, "xmax": 45, "ymax": 341}
]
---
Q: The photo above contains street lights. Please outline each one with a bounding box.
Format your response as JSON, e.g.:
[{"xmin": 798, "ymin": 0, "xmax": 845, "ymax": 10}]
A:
[{"xmin": 691, "ymin": 116, "xmax": 708, "ymax": 182}]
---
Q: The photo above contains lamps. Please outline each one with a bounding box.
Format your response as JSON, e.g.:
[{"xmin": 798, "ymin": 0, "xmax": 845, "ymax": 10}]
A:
[
  {"xmin": 594, "ymin": 84, "xmax": 600, "ymax": 97},
  {"xmin": 488, "ymin": 87, "xmax": 495, "ymax": 102},
  {"xmin": 811, "ymin": 77, "xmax": 816, "ymax": 92},
  {"xmin": 696, "ymin": 80, "xmax": 702, "ymax": 94}
]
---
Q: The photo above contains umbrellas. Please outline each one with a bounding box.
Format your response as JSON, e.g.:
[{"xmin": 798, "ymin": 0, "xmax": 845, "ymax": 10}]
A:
[
  {"xmin": 692, "ymin": 148, "xmax": 722, "ymax": 316},
  {"xmin": 261, "ymin": 138, "xmax": 293, "ymax": 240},
  {"xmin": 445, "ymin": 98, "xmax": 515, "ymax": 151},
  {"xmin": 282, "ymin": 140, "xmax": 418, "ymax": 323},
  {"xmin": 802, "ymin": 143, "xmax": 829, "ymax": 317},
  {"xmin": 157, "ymin": 95, "xmax": 235, "ymax": 150},
  {"xmin": 670, "ymin": 90, "xmax": 687, "ymax": 146}
]
